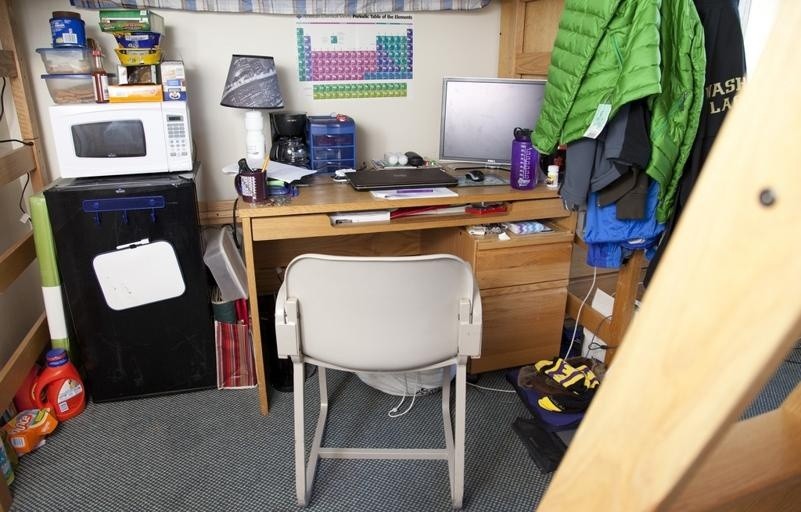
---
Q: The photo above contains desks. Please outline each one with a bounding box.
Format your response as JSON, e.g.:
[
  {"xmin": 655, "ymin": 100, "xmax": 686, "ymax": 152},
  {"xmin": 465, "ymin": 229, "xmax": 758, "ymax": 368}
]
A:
[{"xmin": 236, "ymin": 172, "xmax": 576, "ymax": 416}]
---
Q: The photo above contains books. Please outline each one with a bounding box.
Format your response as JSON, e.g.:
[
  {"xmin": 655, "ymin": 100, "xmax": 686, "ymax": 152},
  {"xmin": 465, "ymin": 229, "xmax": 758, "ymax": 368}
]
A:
[{"xmin": 331, "ymin": 210, "xmax": 391, "ymax": 225}]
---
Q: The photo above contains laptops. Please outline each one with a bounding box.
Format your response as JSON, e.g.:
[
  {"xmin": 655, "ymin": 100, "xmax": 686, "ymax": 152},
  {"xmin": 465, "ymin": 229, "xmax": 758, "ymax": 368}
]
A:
[{"xmin": 344, "ymin": 168, "xmax": 458, "ymax": 192}]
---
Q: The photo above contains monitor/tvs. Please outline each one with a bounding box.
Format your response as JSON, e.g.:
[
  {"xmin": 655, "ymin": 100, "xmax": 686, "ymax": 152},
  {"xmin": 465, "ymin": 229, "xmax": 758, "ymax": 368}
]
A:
[{"xmin": 439, "ymin": 77, "xmax": 547, "ymax": 173}]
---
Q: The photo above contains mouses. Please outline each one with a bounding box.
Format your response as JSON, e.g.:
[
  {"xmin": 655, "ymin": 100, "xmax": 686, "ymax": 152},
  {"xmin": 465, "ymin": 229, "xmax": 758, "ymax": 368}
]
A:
[{"xmin": 464, "ymin": 170, "xmax": 484, "ymax": 182}]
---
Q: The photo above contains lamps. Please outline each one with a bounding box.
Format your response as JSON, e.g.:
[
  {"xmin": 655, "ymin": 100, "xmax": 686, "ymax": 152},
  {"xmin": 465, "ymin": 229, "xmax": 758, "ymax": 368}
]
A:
[{"xmin": 219, "ymin": 54, "xmax": 287, "ymax": 175}]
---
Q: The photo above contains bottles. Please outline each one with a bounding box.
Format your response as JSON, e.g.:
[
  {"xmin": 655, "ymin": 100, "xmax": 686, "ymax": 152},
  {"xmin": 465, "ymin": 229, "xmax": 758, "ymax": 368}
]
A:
[
  {"xmin": 509, "ymin": 126, "xmax": 541, "ymax": 190},
  {"xmin": 547, "ymin": 165, "xmax": 561, "ymax": 191},
  {"xmin": 555, "ymin": 143, "xmax": 566, "ymax": 186},
  {"xmin": 0, "ymin": 427, "xmax": 21, "ymax": 512},
  {"xmin": 91, "ymin": 49, "xmax": 110, "ymax": 103},
  {"xmin": 385, "ymin": 149, "xmax": 425, "ymax": 166}
]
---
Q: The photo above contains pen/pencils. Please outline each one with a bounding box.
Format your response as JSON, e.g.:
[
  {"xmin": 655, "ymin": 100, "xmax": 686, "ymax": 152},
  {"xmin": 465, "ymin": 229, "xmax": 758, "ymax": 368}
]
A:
[
  {"xmin": 396, "ymin": 189, "xmax": 433, "ymax": 193},
  {"xmin": 295, "ymin": 184, "xmax": 310, "ymax": 187},
  {"xmin": 261, "ymin": 155, "xmax": 269, "ymax": 174}
]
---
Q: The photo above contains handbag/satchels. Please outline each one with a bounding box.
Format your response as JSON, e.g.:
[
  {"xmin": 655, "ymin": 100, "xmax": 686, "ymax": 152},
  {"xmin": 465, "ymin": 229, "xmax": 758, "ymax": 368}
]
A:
[{"xmin": 213, "ymin": 299, "xmax": 256, "ymax": 392}]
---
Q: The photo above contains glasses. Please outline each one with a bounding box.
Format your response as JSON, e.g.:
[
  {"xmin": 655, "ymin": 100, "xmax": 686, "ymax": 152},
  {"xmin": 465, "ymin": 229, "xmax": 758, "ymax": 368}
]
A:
[{"xmin": 249, "ymin": 195, "xmax": 286, "ymax": 208}]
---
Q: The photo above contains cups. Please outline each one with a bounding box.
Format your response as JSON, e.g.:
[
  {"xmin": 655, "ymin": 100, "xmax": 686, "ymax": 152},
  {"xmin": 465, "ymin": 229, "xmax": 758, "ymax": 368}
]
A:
[{"xmin": 235, "ymin": 168, "xmax": 267, "ymax": 204}]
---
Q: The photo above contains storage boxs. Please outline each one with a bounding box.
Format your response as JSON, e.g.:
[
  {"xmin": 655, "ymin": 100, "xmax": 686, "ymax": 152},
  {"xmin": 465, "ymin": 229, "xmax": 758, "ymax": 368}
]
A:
[
  {"xmin": 39, "ymin": 72, "xmax": 104, "ymax": 105},
  {"xmin": 35, "ymin": 41, "xmax": 102, "ymax": 73}
]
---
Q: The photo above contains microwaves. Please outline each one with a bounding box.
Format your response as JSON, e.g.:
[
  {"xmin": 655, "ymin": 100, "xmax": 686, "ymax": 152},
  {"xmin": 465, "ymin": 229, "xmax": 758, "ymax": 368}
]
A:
[{"xmin": 45, "ymin": 101, "xmax": 197, "ymax": 180}]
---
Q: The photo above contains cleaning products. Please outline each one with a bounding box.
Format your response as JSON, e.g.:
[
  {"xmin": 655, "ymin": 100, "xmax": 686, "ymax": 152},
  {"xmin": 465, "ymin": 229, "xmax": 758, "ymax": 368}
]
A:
[
  {"xmin": 0, "ymin": 364, "xmax": 57, "ymax": 485},
  {"xmin": 35, "ymin": 349, "xmax": 85, "ymax": 421}
]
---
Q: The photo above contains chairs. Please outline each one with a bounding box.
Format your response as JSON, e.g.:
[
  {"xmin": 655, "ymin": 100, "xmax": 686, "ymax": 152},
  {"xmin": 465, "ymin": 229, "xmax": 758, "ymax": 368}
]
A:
[{"xmin": 272, "ymin": 254, "xmax": 484, "ymax": 509}]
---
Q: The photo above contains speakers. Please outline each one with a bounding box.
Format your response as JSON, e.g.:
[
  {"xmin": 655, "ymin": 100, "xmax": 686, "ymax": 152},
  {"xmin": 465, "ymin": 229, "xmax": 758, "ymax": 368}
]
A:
[{"xmin": 269, "ymin": 111, "xmax": 311, "ymax": 186}]
